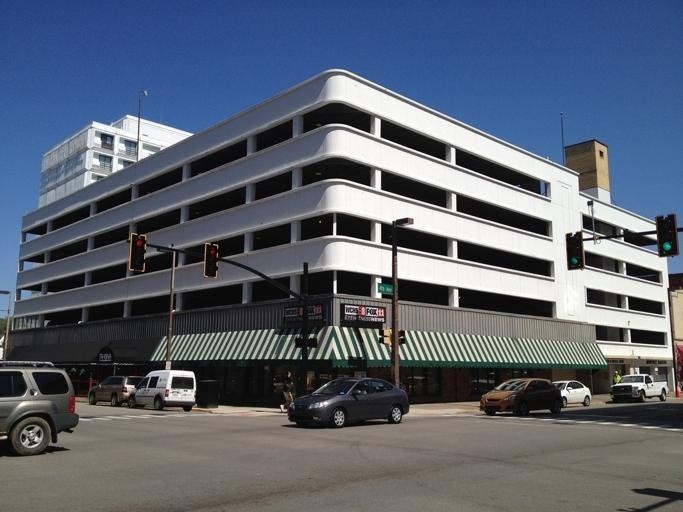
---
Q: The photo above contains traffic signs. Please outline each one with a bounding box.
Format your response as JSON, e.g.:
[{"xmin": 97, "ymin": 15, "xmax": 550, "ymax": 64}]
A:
[{"xmin": 378, "ymin": 282, "xmax": 394, "ymax": 295}]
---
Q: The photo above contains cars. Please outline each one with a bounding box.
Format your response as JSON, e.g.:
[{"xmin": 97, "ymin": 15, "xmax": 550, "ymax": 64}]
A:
[
  {"xmin": 552, "ymin": 380, "xmax": 591, "ymax": 409},
  {"xmin": 285, "ymin": 376, "xmax": 409, "ymax": 427},
  {"xmin": 399, "ymin": 376, "xmax": 440, "ymax": 395},
  {"xmin": 472, "ymin": 373, "xmax": 498, "ymax": 392},
  {"xmin": 479, "ymin": 378, "xmax": 563, "ymax": 415}
]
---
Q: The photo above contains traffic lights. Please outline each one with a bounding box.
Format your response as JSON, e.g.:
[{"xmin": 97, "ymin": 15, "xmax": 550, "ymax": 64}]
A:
[
  {"xmin": 566, "ymin": 231, "xmax": 584, "ymax": 269},
  {"xmin": 128, "ymin": 235, "xmax": 146, "ymax": 272},
  {"xmin": 378, "ymin": 329, "xmax": 391, "ymax": 345},
  {"xmin": 656, "ymin": 213, "xmax": 677, "ymax": 256},
  {"xmin": 397, "ymin": 330, "xmax": 406, "ymax": 344},
  {"xmin": 204, "ymin": 243, "xmax": 221, "ymax": 278}
]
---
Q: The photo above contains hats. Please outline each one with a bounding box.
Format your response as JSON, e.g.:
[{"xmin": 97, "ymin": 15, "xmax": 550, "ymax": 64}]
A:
[{"xmin": 614, "ymin": 371, "xmax": 618, "ymax": 374}]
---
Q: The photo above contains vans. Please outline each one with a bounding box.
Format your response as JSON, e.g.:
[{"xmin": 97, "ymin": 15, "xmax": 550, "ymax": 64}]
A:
[{"xmin": 126, "ymin": 369, "xmax": 196, "ymax": 411}]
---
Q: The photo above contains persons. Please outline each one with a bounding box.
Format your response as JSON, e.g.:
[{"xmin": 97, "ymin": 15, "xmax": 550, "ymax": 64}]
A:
[
  {"xmin": 613, "ymin": 370, "xmax": 621, "ymax": 384},
  {"xmin": 279, "ymin": 371, "xmax": 294, "ymax": 412}
]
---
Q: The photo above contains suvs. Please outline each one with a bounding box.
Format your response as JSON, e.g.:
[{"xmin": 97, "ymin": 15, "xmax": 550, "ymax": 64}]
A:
[
  {"xmin": 86, "ymin": 374, "xmax": 144, "ymax": 405},
  {"xmin": 0, "ymin": 359, "xmax": 79, "ymax": 456}
]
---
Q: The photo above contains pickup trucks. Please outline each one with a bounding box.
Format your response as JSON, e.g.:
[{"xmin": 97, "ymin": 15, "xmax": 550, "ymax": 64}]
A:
[{"xmin": 608, "ymin": 373, "xmax": 668, "ymax": 403}]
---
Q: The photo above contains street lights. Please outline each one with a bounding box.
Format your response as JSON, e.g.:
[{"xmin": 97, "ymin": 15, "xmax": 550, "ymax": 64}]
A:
[
  {"xmin": 0, "ymin": 289, "xmax": 12, "ymax": 361},
  {"xmin": 389, "ymin": 215, "xmax": 414, "ymax": 391},
  {"xmin": 157, "ymin": 242, "xmax": 175, "ymax": 368}
]
